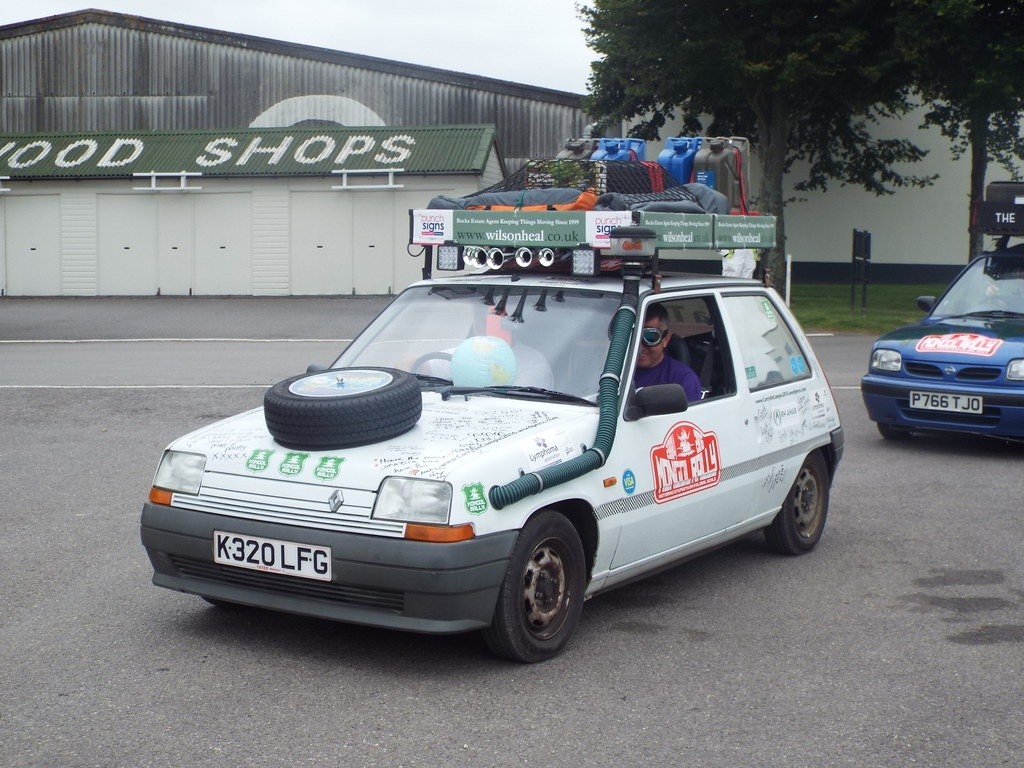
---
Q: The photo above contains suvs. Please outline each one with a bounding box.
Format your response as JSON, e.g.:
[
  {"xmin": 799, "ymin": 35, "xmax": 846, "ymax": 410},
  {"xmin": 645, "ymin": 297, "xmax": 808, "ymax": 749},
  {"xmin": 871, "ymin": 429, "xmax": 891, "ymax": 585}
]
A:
[
  {"xmin": 140, "ymin": 224, "xmax": 847, "ymax": 664},
  {"xmin": 859, "ymin": 236, "xmax": 1024, "ymax": 448}
]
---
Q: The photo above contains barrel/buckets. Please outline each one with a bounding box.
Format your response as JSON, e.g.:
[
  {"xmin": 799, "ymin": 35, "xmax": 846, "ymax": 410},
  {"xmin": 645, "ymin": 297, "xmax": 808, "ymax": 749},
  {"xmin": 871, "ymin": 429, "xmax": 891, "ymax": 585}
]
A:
[
  {"xmin": 690, "ymin": 136, "xmax": 752, "ymax": 213},
  {"xmin": 657, "ymin": 136, "xmax": 702, "ymax": 190},
  {"xmin": 556, "ymin": 137, "xmax": 646, "ymax": 168}
]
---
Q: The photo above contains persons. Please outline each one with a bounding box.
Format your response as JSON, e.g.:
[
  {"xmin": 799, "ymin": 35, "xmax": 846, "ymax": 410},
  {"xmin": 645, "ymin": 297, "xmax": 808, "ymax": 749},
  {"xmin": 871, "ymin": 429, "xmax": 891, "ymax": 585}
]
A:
[
  {"xmin": 633, "ymin": 302, "xmax": 703, "ymax": 412},
  {"xmin": 429, "ymin": 293, "xmax": 555, "ymax": 399},
  {"xmin": 985, "ymin": 283, "xmax": 999, "ymax": 297}
]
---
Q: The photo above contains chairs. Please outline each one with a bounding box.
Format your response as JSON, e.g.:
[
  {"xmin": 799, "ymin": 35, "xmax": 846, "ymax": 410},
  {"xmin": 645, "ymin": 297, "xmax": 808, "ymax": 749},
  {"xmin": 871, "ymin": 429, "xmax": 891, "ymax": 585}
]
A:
[{"xmin": 665, "ymin": 332, "xmax": 690, "ymax": 367}]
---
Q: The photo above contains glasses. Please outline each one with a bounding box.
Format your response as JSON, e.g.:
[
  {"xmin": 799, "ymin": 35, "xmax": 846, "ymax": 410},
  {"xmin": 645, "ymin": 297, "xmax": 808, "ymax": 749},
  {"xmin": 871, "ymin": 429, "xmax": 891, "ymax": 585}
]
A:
[{"xmin": 641, "ymin": 328, "xmax": 668, "ymax": 347}]
